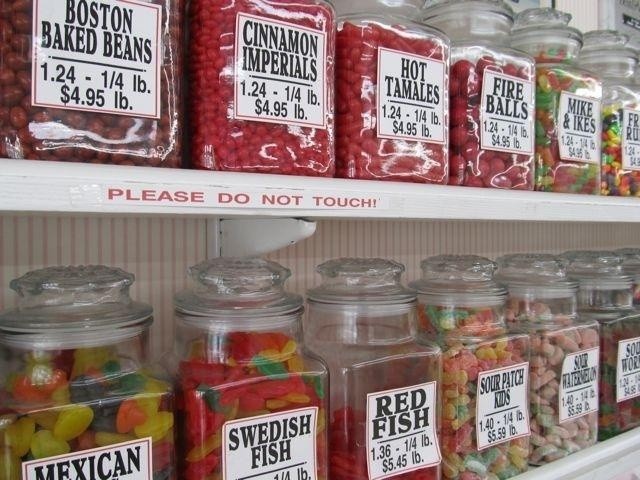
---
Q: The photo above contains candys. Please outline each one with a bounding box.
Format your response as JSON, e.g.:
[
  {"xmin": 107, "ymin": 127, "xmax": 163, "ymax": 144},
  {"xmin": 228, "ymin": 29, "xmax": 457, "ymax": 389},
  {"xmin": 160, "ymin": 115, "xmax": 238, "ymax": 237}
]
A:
[
  {"xmin": 504, "ymin": 296, "xmax": 600, "ymax": 464},
  {"xmin": 335, "ymin": 22, "xmax": 450, "ymax": 186},
  {"xmin": 184, "ymin": 1, "xmax": 338, "ymax": 179},
  {"xmin": 416, "ymin": 304, "xmax": 530, "ymax": 480},
  {"xmin": 449, "ymin": 55, "xmax": 535, "ymax": 191},
  {"xmin": 597, "ymin": 316, "xmax": 639, "ymax": 440},
  {"xmin": 2, "ymin": 0, "xmax": 186, "ymax": 169},
  {"xmin": 328, "ymin": 407, "xmax": 438, "ymax": 479},
  {"xmin": 0, "ymin": 345, "xmax": 178, "ymax": 480},
  {"xmin": 601, "ymin": 102, "xmax": 639, "ymax": 197},
  {"xmin": 534, "ymin": 47, "xmax": 603, "ymax": 195},
  {"xmin": 172, "ymin": 331, "xmax": 328, "ymax": 480}
]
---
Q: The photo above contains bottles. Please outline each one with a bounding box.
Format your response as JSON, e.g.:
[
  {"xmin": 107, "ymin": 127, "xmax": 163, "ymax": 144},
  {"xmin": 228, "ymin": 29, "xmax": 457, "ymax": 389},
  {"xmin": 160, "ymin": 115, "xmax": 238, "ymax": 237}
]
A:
[
  {"xmin": 407, "ymin": 254, "xmax": 531, "ymax": 480},
  {"xmin": 338, "ymin": 0, "xmax": 452, "ymax": 183},
  {"xmin": 3, "ymin": 264, "xmax": 177, "ymax": 479},
  {"xmin": 166, "ymin": 257, "xmax": 332, "ymax": 480},
  {"xmin": 2, "ymin": 0, "xmax": 186, "ymax": 165},
  {"xmin": 303, "ymin": 258, "xmax": 443, "ymax": 480},
  {"xmin": 577, "ymin": 30, "xmax": 638, "ymax": 190},
  {"xmin": 491, "ymin": 250, "xmax": 601, "ymax": 465},
  {"xmin": 508, "ymin": 9, "xmax": 603, "ymax": 193},
  {"xmin": 559, "ymin": 245, "xmax": 640, "ymax": 441},
  {"xmin": 420, "ymin": 1, "xmax": 538, "ymax": 190},
  {"xmin": 190, "ymin": 1, "xmax": 341, "ymax": 177}
]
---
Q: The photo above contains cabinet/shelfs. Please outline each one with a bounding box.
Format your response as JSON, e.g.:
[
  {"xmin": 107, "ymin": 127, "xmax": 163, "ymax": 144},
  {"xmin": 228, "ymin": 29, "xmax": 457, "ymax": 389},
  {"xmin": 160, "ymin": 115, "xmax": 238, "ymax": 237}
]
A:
[{"xmin": 0, "ymin": 157, "xmax": 640, "ymax": 480}]
